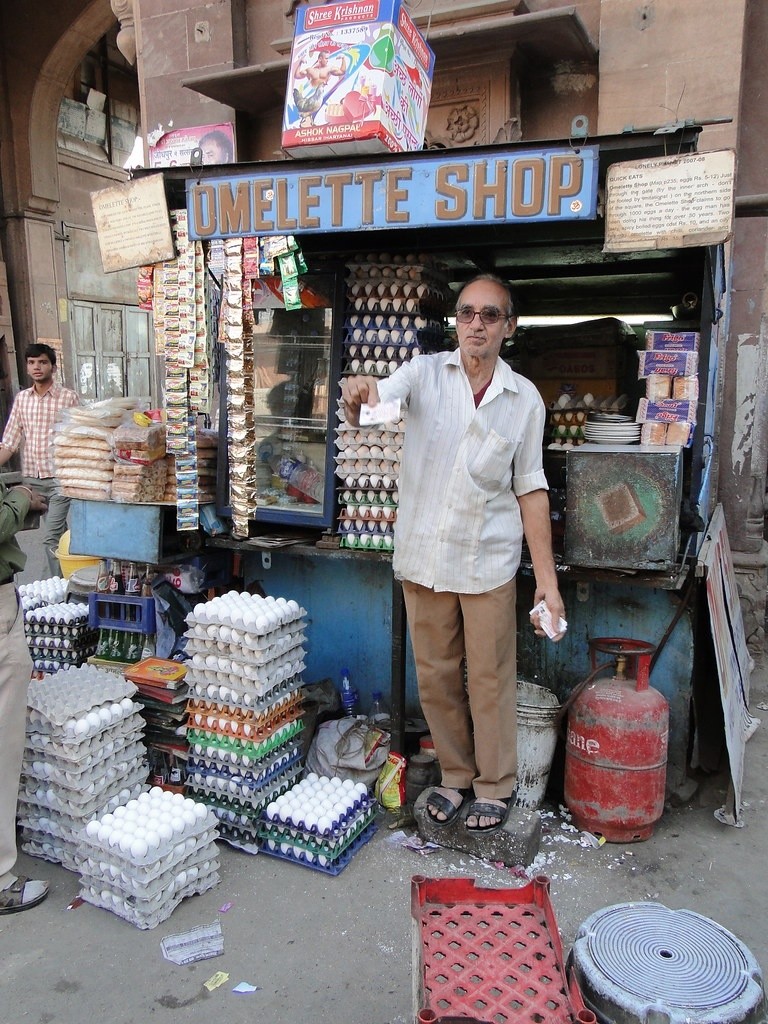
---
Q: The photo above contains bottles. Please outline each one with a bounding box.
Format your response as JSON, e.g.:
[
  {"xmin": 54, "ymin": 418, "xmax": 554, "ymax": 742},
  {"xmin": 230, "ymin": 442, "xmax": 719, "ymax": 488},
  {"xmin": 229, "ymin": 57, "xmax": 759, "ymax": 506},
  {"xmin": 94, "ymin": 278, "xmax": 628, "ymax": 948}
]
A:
[
  {"xmin": 259, "ymin": 450, "xmax": 325, "ymax": 505},
  {"xmin": 308, "ymin": 355, "xmax": 328, "ymax": 429},
  {"xmin": 91, "ymin": 627, "xmax": 156, "ymax": 665},
  {"xmin": 94, "ymin": 557, "xmax": 159, "ymax": 598},
  {"xmin": 142, "ymin": 746, "xmax": 188, "ymax": 788},
  {"xmin": 368, "ymin": 691, "xmax": 391, "ymax": 736},
  {"xmin": 336, "ymin": 666, "xmax": 361, "ymax": 719}
]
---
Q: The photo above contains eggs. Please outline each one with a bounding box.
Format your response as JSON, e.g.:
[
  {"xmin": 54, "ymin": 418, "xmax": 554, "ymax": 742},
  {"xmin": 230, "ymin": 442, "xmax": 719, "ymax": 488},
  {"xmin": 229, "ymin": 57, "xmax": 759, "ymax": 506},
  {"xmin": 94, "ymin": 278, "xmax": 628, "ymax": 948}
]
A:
[
  {"xmin": 14, "ymin": 577, "xmax": 373, "ymax": 919},
  {"xmin": 335, "ymin": 251, "xmax": 446, "ymax": 549},
  {"xmin": 547, "ymin": 393, "xmax": 595, "ymax": 452}
]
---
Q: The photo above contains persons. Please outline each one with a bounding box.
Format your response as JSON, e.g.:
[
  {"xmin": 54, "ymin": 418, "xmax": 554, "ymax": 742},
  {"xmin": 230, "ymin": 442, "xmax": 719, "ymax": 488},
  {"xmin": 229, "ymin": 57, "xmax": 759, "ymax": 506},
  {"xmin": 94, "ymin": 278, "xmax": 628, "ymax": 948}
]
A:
[
  {"xmin": 254, "ymin": 380, "xmax": 324, "ymax": 506},
  {"xmin": 198, "ymin": 130, "xmax": 233, "ymax": 165},
  {"xmin": 0, "ymin": 343, "xmax": 80, "ymax": 577},
  {"xmin": 343, "ymin": 273, "xmax": 565, "ymax": 832},
  {"xmin": 294, "ymin": 50, "xmax": 347, "ymax": 87},
  {"xmin": 0, "ymin": 476, "xmax": 50, "ymax": 915}
]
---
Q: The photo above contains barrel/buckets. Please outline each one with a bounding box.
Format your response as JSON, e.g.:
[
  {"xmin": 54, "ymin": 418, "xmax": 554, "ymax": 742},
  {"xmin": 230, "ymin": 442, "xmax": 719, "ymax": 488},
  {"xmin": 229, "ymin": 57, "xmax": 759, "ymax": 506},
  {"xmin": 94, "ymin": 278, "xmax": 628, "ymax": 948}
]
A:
[
  {"xmin": 49, "ymin": 525, "xmax": 103, "ymax": 579},
  {"xmin": 510, "ymin": 677, "xmax": 559, "ymax": 813}
]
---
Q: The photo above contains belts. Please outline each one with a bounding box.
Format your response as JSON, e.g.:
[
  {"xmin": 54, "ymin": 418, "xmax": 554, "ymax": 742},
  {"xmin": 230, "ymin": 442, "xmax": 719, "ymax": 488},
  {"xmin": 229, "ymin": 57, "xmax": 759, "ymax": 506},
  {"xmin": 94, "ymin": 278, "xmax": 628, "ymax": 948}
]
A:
[{"xmin": 0, "ymin": 573, "xmax": 14, "ymax": 585}]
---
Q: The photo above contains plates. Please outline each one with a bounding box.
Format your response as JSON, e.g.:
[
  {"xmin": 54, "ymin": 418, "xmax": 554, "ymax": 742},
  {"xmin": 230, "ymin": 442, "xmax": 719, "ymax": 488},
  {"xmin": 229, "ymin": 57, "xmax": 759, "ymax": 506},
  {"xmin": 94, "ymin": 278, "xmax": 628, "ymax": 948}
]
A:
[{"xmin": 584, "ymin": 411, "xmax": 641, "ymax": 444}]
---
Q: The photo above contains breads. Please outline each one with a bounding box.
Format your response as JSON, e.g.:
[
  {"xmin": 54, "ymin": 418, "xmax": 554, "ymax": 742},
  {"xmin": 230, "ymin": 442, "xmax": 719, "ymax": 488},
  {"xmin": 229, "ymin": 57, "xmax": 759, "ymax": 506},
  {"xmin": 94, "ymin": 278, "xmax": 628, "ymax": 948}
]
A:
[{"xmin": 634, "ymin": 330, "xmax": 700, "ymax": 448}]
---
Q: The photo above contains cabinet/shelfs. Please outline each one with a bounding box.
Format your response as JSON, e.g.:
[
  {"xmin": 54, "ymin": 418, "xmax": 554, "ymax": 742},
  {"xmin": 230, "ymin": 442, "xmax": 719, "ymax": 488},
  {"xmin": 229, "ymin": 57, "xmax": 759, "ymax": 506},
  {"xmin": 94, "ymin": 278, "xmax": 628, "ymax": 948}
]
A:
[{"xmin": 213, "ymin": 262, "xmax": 349, "ymax": 528}]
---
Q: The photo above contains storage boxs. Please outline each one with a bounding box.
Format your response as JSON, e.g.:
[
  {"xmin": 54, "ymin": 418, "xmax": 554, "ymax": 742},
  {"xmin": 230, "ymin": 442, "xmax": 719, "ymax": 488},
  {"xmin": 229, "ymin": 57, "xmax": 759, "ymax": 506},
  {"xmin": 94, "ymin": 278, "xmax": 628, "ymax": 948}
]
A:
[
  {"xmin": 279, "ymin": 0, "xmax": 436, "ymax": 157},
  {"xmin": 66, "ymin": 497, "xmax": 227, "ymax": 565},
  {"xmin": 88, "ymin": 590, "xmax": 157, "ymax": 634}
]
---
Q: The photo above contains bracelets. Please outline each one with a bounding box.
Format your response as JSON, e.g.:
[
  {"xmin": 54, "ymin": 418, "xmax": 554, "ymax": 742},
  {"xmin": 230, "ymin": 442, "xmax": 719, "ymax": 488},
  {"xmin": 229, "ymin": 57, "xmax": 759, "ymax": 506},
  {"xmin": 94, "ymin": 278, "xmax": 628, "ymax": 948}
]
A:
[{"xmin": 15, "ymin": 486, "xmax": 33, "ymax": 501}]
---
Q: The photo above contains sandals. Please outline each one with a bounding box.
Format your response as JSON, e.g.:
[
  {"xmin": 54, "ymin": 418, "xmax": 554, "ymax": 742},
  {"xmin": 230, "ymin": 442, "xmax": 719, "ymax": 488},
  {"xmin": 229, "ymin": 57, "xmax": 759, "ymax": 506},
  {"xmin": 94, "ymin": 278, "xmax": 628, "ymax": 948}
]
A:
[
  {"xmin": 0, "ymin": 875, "xmax": 49, "ymax": 914},
  {"xmin": 424, "ymin": 784, "xmax": 475, "ymax": 830},
  {"xmin": 463, "ymin": 790, "xmax": 518, "ymax": 836}
]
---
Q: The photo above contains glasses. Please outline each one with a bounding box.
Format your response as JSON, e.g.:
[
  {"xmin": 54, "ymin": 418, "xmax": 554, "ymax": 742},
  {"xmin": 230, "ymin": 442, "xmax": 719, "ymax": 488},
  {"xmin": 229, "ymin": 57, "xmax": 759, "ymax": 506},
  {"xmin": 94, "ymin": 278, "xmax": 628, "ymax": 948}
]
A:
[{"xmin": 454, "ymin": 306, "xmax": 512, "ymax": 324}]
